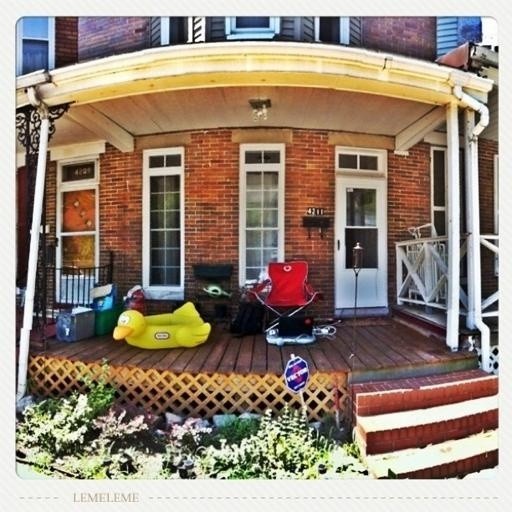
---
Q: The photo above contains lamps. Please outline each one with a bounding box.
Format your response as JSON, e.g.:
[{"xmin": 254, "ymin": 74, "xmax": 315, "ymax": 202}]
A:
[
  {"xmin": 351, "ymin": 240, "xmax": 364, "ymax": 370},
  {"xmin": 249, "ymin": 99, "xmax": 271, "ymax": 123}
]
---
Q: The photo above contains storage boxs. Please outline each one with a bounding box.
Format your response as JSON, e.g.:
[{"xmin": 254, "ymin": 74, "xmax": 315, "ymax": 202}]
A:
[
  {"xmin": 95, "ymin": 304, "xmax": 123, "ymax": 336},
  {"xmin": 56, "ymin": 311, "xmax": 95, "ymax": 342}
]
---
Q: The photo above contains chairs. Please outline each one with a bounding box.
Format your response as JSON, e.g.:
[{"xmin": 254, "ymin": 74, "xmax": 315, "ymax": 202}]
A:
[
  {"xmin": 248, "ymin": 261, "xmax": 319, "ymax": 334},
  {"xmin": 193, "ymin": 265, "xmax": 232, "ymax": 329}
]
